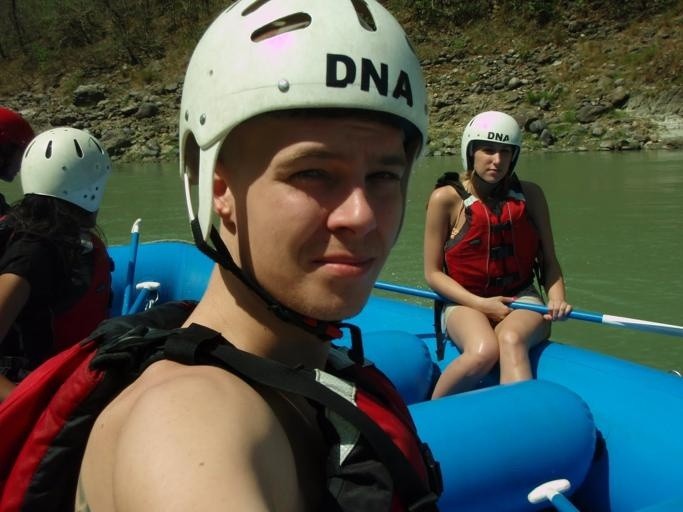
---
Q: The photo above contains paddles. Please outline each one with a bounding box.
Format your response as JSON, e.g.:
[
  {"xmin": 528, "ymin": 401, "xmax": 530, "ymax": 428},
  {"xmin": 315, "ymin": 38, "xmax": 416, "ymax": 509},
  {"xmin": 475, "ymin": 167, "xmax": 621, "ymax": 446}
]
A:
[{"xmin": 371, "ymin": 280, "xmax": 683, "ymax": 339}]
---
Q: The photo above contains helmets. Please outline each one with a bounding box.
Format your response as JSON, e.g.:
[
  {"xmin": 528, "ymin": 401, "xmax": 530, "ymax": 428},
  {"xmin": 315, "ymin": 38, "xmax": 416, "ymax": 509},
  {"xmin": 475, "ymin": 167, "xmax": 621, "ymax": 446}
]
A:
[
  {"xmin": 178, "ymin": 1, "xmax": 429, "ymax": 248},
  {"xmin": 460, "ymin": 110, "xmax": 523, "ymax": 178},
  {"xmin": 0, "ymin": 106, "xmax": 35, "ymax": 150},
  {"xmin": 20, "ymin": 126, "xmax": 114, "ymax": 213}
]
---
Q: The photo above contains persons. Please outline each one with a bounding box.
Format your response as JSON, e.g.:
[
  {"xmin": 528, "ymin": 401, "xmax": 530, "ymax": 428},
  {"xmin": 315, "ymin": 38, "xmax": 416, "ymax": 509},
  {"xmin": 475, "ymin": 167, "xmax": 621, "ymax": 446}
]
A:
[
  {"xmin": 0, "ymin": 126, "xmax": 117, "ymax": 405},
  {"xmin": 422, "ymin": 109, "xmax": 573, "ymax": 401},
  {"xmin": 70, "ymin": 0, "xmax": 442, "ymax": 512},
  {"xmin": 1, "ymin": 105, "xmax": 35, "ymax": 218}
]
